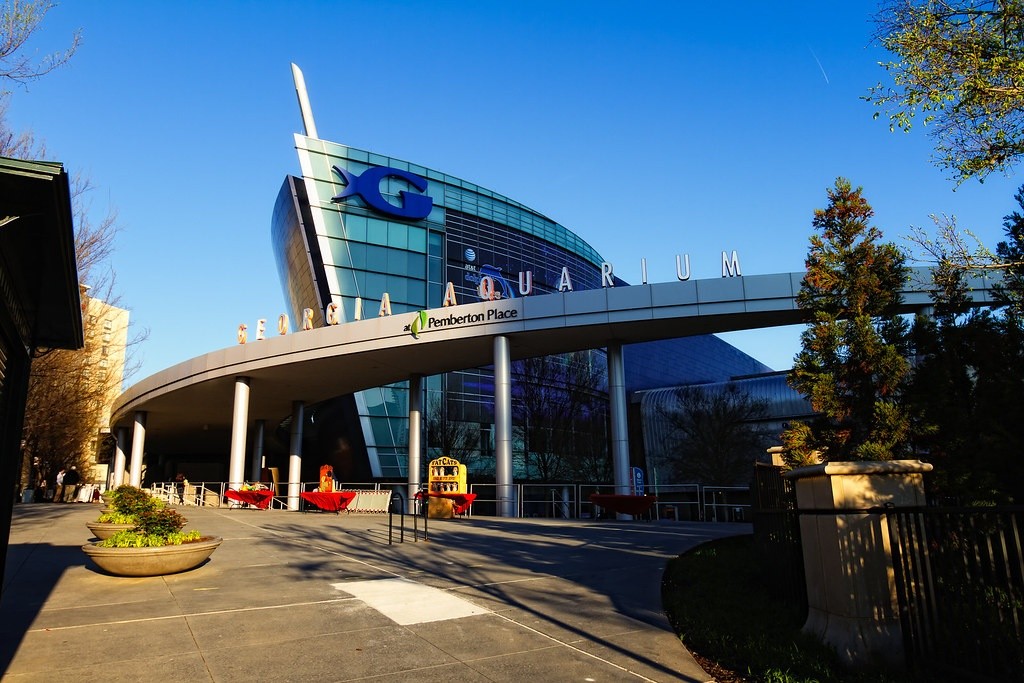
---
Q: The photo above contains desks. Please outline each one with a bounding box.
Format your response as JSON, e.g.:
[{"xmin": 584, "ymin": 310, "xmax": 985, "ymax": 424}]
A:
[
  {"xmin": 414, "ymin": 493, "xmax": 476, "ymax": 520},
  {"xmin": 590, "ymin": 493, "xmax": 658, "ymax": 524},
  {"xmin": 225, "ymin": 488, "xmax": 275, "ymax": 511},
  {"xmin": 300, "ymin": 491, "xmax": 357, "ymax": 515}
]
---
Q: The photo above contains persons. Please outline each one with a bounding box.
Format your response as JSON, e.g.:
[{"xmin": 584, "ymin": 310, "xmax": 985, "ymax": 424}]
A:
[
  {"xmin": 55, "ymin": 470, "xmax": 67, "ymax": 502},
  {"xmin": 176, "ymin": 473, "xmax": 184, "ymax": 505},
  {"xmin": 93, "ymin": 489, "xmax": 99, "ymax": 503},
  {"xmin": 33, "ymin": 480, "xmax": 48, "ymax": 502}
]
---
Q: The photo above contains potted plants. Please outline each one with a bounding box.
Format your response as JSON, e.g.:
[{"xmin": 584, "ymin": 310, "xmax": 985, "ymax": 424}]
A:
[{"xmin": 82, "ymin": 486, "xmax": 224, "ymax": 577}]
